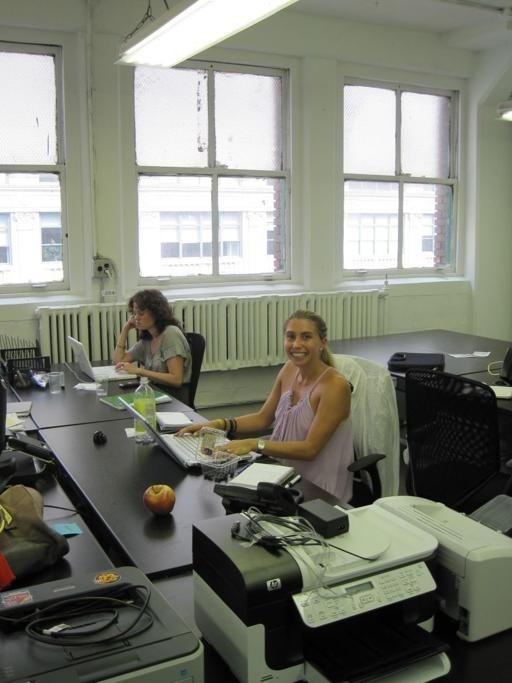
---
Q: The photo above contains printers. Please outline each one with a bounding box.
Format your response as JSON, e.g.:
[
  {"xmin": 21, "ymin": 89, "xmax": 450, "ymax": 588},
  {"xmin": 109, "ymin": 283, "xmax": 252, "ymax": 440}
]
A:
[
  {"xmin": 192, "ymin": 504, "xmax": 456, "ymax": 682},
  {"xmin": 0, "ymin": 566, "xmax": 204, "ymax": 683},
  {"xmin": 374, "ymin": 492, "xmax": 510, "ymax": 644}
]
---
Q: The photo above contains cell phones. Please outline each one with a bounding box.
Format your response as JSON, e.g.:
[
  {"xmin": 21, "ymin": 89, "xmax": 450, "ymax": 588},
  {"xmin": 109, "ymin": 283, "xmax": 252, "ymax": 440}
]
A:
[{"xmin": 119, "ymin": 381, "xmax": 140, "ymax": 388}]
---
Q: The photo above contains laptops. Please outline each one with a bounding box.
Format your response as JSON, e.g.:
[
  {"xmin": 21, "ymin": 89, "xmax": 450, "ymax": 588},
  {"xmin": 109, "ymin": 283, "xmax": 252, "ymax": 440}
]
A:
[
  {"xmin": 68, "ymin": 335, "xmax": 138, "ymax": 382},
  {"xmin": 118, "ymin": 396, "xmax": 252, "ymax": 468}
]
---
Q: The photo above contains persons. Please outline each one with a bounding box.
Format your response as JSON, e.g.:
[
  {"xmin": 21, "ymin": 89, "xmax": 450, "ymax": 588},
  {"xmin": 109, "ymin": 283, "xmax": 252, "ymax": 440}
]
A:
[
  {"xmin": 112, "ymin": 289, "xmax": 192, "ymax": 406},
  {"xmin": 174, "ymin": 308, "xmax": 354, "ymax": 503}
]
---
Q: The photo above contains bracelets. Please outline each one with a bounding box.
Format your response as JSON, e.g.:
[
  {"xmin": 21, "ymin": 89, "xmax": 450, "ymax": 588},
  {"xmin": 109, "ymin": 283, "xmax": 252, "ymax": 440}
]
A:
[
  {"xmin": 216, "ymin": 418, "xmax": 237, "ymax": 432},
  {"xmin": 116, "ymin": 344, "xmax": 126, "ymax": 349}
]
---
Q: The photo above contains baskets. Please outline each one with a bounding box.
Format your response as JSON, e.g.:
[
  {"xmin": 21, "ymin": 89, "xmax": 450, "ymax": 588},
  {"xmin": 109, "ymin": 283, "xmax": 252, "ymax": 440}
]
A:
[{"xmin": 0, "ymin": 346, "xmax": 51, "ymax": 386}]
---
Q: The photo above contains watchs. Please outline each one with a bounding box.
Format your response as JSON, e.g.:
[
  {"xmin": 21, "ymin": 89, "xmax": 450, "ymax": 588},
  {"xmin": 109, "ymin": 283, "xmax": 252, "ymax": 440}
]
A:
[{"xmin": 257, "ymin": 436, "xmax": 264, "ymax": 454}]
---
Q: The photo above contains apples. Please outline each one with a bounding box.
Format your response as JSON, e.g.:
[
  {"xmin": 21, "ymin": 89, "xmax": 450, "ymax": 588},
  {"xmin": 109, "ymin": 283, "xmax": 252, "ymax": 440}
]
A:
[{"xmin": 144, "ymin": 484, "xmax": 176, "ymax": 515}]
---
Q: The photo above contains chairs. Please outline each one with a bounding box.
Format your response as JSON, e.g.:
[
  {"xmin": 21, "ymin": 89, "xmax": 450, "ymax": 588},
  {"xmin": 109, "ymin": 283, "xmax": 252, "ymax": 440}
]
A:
[
  {"xmin": 402, "ymin": 368, "xmax": 501, "ymax": 507},
  {"xmin": 179, "ymin": 332, "xmax": 207, "ymax": 410},
  {"xmin": 323, "ymin": 354, "xmax": 402, "ymax": 500}
]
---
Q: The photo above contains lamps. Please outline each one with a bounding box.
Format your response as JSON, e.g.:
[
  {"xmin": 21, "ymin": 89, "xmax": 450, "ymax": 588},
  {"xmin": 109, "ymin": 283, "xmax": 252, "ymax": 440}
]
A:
[{"xmin": 497, "ymin": 94, "xmax": 511, "ymax": 122}]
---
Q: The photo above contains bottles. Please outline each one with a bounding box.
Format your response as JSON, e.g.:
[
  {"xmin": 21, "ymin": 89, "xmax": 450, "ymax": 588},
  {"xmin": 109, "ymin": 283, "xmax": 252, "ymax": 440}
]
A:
[{"xmin": 133, "ymin": 377, "xmax": 158, "ymax": 443}]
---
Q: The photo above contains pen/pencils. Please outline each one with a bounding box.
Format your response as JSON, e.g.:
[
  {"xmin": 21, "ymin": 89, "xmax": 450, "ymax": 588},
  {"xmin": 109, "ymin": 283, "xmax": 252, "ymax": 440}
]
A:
[{"xmin": 284, "ymin": 475, "xmax": 301, "ymax": 488}]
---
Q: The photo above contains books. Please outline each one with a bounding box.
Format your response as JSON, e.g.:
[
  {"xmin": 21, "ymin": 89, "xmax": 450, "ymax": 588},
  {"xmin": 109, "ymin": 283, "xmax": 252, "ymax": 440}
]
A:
[
  {"xmin": 99, "ymin": 389, "xmax": 169, "ymax": 410},
  {"xmin": 227, "ymin": 463, "xmax": 295, "ymax": 490}
]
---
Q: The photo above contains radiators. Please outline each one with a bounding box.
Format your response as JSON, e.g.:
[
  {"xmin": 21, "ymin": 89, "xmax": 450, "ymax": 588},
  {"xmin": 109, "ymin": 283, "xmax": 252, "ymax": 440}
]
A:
[{"xmin": 34, "ymin": 288, "xmax": 381, "ymax": 374}]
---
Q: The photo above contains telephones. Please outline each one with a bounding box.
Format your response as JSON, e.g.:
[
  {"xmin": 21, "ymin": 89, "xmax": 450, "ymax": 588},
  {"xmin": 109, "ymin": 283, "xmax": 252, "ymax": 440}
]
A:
[{"xmin": 214, "ymin": 482, "xmax": 303, "ymax": 516}]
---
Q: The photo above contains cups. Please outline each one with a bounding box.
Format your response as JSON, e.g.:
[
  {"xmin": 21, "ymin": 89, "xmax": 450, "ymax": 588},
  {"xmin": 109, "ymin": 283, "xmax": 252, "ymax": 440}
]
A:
[
  {"xmin": 95, "ymin": 373, "xmax": 108, "ymax": 397},
  {"xmin": 48, "ymin": 371, "xmax": 63, "ymax": 394}
]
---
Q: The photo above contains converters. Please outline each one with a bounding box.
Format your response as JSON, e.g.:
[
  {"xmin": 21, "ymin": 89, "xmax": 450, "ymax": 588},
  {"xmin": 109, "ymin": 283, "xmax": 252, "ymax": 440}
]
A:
[{"xmin": 299, "ymin": 497, "xmax": 349, "ymax": 539}]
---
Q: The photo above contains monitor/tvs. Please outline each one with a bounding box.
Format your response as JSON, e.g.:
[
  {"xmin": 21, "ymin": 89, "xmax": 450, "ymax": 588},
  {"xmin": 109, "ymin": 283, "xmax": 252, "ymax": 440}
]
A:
[{"xmin": 0, "ymin": 380, "xmax": 55, "ymax": 481}]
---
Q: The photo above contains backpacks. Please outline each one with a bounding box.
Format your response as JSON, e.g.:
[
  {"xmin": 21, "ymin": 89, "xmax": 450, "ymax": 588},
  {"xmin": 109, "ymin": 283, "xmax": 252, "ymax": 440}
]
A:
[{"xmin": 0, "ymin": 482, "xmax": 70, "ymax": 593}]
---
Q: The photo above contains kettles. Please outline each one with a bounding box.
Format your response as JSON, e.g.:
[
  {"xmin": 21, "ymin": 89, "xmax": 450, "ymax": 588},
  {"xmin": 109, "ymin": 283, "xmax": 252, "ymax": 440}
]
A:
[{"xmin": 495, "ymin": 343, "xmax": 512, "ymax": 385}]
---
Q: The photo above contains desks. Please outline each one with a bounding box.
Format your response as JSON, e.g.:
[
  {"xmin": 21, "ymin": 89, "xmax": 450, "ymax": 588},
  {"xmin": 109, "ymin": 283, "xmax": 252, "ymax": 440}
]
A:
[{"xmin": 326, "ymin": 330, "xmax": 511, "ymax": 422}]
